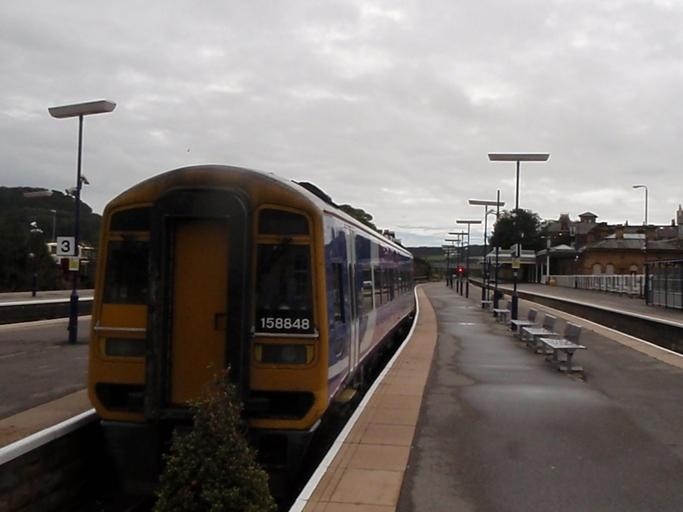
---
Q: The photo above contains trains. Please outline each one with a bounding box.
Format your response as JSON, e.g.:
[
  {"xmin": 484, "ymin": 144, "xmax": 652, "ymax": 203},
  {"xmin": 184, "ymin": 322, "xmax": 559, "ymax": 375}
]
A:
[{"xmin": 84, "ymin": 164, "xmax": 413, "ymax": 445}]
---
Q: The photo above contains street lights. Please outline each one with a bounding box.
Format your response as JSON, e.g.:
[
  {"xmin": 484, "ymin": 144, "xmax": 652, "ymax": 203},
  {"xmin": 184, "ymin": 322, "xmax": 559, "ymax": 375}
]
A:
[
  {"xmin": 468, "ymin": 200, "xmax": 504, "ymax": 309},
  {"xmin": 23, "ymin": 190, "xmax": 53, "ymax": 298},
  {"xmin": 440, "ymin": 220, "xmax": 482, "ymax": 297},
  {"xmin": 632, "ymin": 186, "xmax": 647, "ymax": 225},
  {"xmin": 487, "ymin": 153, "xmax": 551, "ymax": 331},
  {"xmin": 47, "ymin": 101, "xmax": 117, "ymax": 346}
]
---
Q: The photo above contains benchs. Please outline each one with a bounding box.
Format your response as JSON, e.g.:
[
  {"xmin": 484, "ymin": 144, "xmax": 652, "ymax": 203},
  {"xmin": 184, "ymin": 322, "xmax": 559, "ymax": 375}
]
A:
[{"xmin": 493, "ymin": 300, "xmax": 587, "ymax": 373}]
